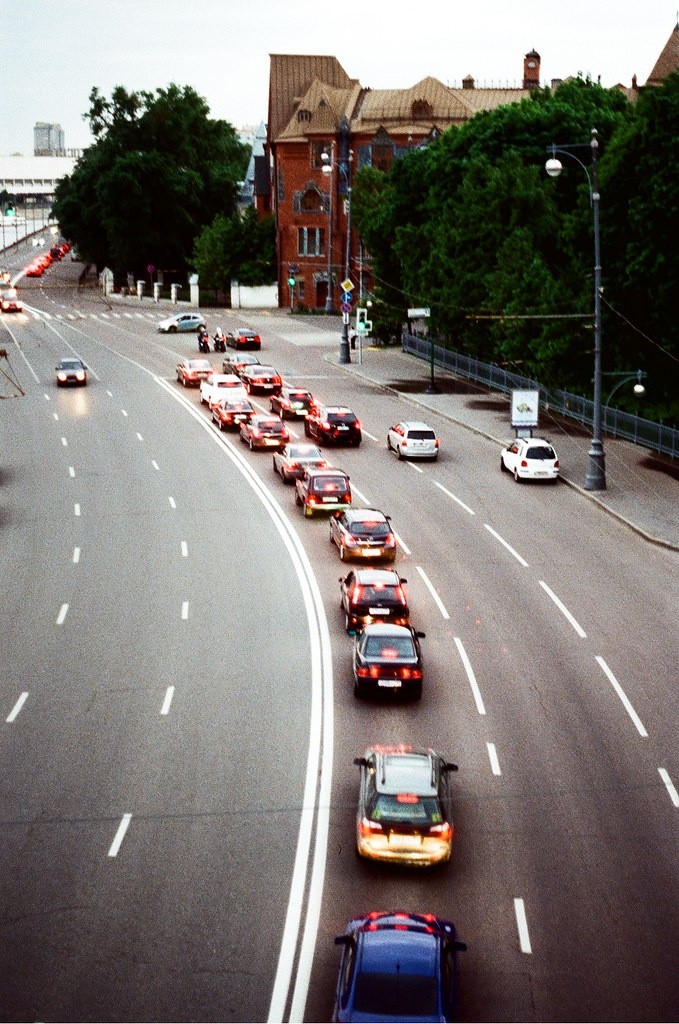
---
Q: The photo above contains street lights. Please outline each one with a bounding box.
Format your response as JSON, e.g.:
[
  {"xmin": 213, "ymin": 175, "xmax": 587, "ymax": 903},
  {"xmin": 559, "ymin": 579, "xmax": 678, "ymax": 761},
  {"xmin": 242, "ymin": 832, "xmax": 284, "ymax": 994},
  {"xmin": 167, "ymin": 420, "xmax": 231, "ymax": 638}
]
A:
[
  {"xmin": 544, "ymin": 143, "xmax": 609, "ymax": 493},
  {"xmin": 318, "ymin": 150, "xmax": 352, "ymax": 364}
]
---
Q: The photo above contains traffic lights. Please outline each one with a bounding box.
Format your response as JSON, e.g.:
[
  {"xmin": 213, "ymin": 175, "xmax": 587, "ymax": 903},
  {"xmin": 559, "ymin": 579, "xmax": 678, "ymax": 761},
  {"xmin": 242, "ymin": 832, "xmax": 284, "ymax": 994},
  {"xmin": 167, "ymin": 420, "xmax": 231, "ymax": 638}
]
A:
[
  {"xmin": 357, "ymin": 309, "xmax": 373, "ymax": 334},
  {"xmin": 287, "ymin": 269, "xmax": 296, "ymax": 286},
  {"xmin": 4, "ymin": 201, "xmax": 15, "ymax": 217}
]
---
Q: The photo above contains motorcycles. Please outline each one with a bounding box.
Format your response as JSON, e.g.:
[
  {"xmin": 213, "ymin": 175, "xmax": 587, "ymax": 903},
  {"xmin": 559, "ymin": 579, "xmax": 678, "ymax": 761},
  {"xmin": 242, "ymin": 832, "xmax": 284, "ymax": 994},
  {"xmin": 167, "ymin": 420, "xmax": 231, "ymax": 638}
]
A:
[
  {"xmin": 213, "ymin": 335, "xmax": 227, "ymax": 352},
  {"xmin": 197, "ymin": 335, "xmax": 210, "ymax": 352}
]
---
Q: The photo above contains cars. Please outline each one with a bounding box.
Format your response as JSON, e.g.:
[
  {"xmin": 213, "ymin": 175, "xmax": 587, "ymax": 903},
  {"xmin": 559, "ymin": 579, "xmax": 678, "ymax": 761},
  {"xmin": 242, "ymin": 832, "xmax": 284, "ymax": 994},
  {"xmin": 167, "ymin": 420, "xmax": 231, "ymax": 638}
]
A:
[
  {"xmin": 0, "ymin": 236, "xmax": 73, "ymax": 314},
  {"xmin": 54, "ymin": 358, "xmax": 88, "ymax": 387},
  {"xmin": 500, "ymin": 435, "xmax": 561, "ymax": 483},
  {"xmin": 157, "ymin": 312, "xmax": 207, "ymax": 333},
  {"xmin": 173, "ymin": 353, "xmax": 460, "ymax": 1024},
  {"xmin": 226, "ymin": 329, "xmax": 262, "ymax": 351}
]
